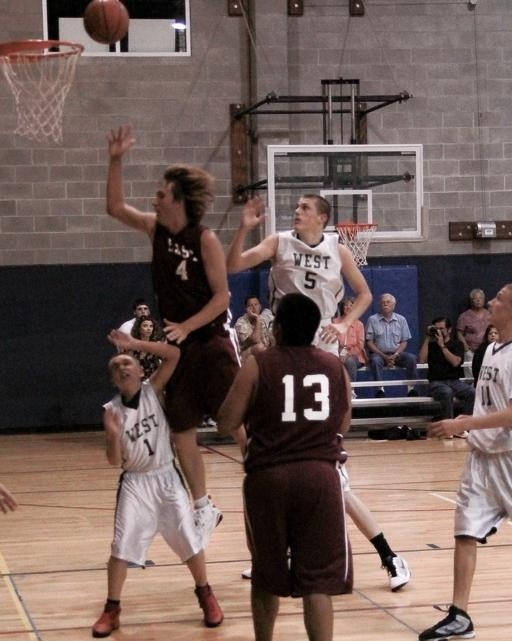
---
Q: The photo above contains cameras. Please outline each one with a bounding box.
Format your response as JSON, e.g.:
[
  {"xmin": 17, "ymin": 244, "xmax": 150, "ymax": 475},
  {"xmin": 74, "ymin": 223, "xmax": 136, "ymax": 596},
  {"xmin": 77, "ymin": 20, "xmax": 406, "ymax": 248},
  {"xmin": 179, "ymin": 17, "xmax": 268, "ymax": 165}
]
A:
[{"xmin": 429, "ymin": 327, "xmax": 439, "ymax": 337}]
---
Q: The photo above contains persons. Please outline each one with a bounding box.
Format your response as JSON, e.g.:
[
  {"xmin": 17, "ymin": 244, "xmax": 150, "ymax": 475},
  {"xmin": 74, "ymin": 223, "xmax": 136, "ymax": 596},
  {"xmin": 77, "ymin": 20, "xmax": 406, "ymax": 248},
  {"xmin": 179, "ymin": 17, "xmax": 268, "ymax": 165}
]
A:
[
  {"xmin": 472, "ymin": 324, "xmax": 499, "ymax": 389},
  {"xmin": 457, "ymin": 288, "xmax": 488, "ymax": 378},
  {"xmin": 332, "ymin": 297, "xmax": 368, "ymax": 400},
  {"xmin": 418, "ymin": 282, "xmax": 512, "ymax": 641},
  {"xmin": 90, "ymin": 330, "xmax": 224, "ymax": 637},
  {"xmin": 419, "ymin": 316, "xmax": 476, "ymax": 439},
  {"xmin": 115, "ymin": 299, "xmax": 151, "ymax": 354},
  {"xmin": 227, "ymin": 193, "xmax": 372, "ymax": 359},
  {"xmin": 106, "ymin": 121, "xmax": 248, "ymax": 548},
  {"xmin": 125, "ymin": 317, "xmax": 162, "ymax": 378},
  {"xmin": 0, "ymin": 483, "xmax": 18, "ymax": 514},
  {"xmin": 234, "ymin": 295, "xmax": 272, "ymax": 358},
  {"xmin": 217, "ymin": 292, "xmax": 353, "ymax": 640},
  {"xmin": 241, "ymin": 457, "xmax": 410, "ymax": 592},
  {"xmin": 365, "ymin": 293, "xmax": 417, "ymax": 397}
]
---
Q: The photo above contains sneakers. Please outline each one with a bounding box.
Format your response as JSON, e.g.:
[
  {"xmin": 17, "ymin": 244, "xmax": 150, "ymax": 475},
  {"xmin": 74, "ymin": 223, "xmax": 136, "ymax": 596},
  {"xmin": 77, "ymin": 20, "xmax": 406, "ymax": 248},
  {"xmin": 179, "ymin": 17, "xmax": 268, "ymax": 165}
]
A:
[
  {"xmin": 92, "ymin": 603, "xmax": 122, "ymax": 638},
  {"xmin": 195, "ymin": 585, "xmax": 225, "ymax": 629},
  {"xmin": 417, "ymin": 604, "xmax": 476, "ymax": 641},
  {"xmin": 381, "ymin": 552, "xmax": 412, "ymax": 591},
  {"xmin": 406, "ymin": 388, "xmax": 419, "ymax": 397},
  {"xmin": 241, "ymin": 553, "xmax": 291, "ymax": 580},
  {"xmin": 192, "ymin": 501, "xmax": 223, "ymax": 549},
  {"xmin": 375, "ymin": 389, "xmax": 385, "ymax": 398}
]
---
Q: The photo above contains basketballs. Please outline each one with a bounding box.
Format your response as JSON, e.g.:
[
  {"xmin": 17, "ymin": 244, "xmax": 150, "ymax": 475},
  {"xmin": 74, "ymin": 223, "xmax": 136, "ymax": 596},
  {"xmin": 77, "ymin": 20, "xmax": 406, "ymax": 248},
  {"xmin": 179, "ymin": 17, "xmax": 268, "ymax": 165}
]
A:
[{"xmin": 83, "ymin": 0, "xmax": 130, "ymax": 44}]
